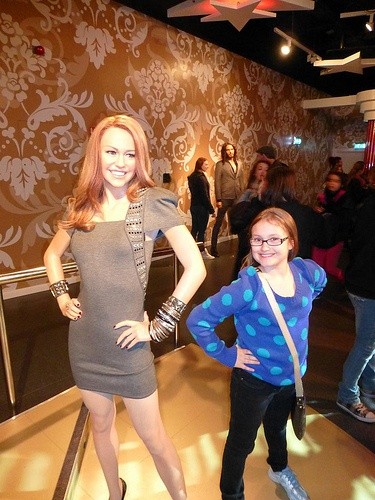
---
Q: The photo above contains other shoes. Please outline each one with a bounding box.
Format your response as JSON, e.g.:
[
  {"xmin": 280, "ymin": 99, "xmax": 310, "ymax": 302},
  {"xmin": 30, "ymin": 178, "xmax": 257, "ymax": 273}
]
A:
[
  {"xmin": 210, "ymin": 251, "xmax": 220, "ymax": 257},
  {"xmin": 199, "ymin": 248, "xmax": 215, "ymax": 260}
]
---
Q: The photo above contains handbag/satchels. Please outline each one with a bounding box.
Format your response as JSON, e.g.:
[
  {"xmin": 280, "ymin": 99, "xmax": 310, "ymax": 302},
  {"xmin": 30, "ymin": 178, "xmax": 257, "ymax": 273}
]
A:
[{"xmin": 290, "ymin": 391, "xmax": 308, "ymax": 440}]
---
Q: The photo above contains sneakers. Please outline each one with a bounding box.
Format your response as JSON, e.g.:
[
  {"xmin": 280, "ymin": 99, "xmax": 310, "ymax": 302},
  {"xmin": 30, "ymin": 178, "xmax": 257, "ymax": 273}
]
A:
[
  {"xmin": 267, "ymin": 464, "xmax": 311, "ymax": 500},
  {"xmin": 336, "ymin": 397, "xmax": 375, "ymax": 422}
]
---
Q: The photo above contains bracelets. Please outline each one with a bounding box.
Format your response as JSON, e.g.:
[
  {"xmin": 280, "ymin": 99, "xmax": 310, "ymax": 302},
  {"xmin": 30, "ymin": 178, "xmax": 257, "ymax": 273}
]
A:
[
  {"xmin": 50, "ymin": 280, "xmax": 69, "ymax": 299},
  {"xmin": 149, "ymin": 296, "xmax": 187, "ymax": 343}
]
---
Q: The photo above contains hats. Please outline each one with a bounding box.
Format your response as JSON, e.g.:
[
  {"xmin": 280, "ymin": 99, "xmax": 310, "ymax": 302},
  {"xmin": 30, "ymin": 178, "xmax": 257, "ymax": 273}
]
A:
[{"xmin": 255, "ymin": 145, "xmax": 277, "ymax": 154}]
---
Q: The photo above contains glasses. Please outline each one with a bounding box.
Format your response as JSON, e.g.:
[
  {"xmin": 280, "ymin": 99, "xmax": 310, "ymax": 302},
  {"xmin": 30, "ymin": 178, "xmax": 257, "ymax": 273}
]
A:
[{"xmin": 250, "ymin": 236, "xmax": 291, "ymax": 247}]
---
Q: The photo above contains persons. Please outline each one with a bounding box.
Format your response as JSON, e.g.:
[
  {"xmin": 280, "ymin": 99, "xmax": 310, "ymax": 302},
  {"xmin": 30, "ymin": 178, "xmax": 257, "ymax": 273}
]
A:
[
  {"xmin": 311, "ymin": 170, "xmax": 345, "ymax": 283},
  {"xmin": 186, "ymin": 208, "xmax": 327, "ymax": 500},
  {"xmin": 188, "ymin": 158, "xmax": 216, "ymax": 243},
  {"xmin": 256, "ymin": 146, "xmax": 288, "ymax": 167},
  {"xmin": 211, "ymin": 143, "xmax": 244, "ymax": 256},
  {"xmin": 230, "ymin": 166, "xmax": 343, "ymax": 280},
  {"xmin": 348, "ymin": 161, "xmax": 366, "ymax": 187},
  {"xmin": 44, "ymin": 115, "xmax": 207, "ymax": 500},
  {"xmin": 336, "ymin": 167, "xmax": 375, "ymax": 422},
  {"xmin": 237, "ymin": 161, "xmax": 270, "ymax": 203},
  {"xmin": 329, "ymin": 157, "xmax": 342, "ymax": 170}
]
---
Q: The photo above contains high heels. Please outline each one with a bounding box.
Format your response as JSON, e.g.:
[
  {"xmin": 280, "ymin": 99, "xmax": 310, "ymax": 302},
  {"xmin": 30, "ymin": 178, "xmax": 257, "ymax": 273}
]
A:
[{"xmin": 119, "ymin": 476, "xmax": 127, "ymax": 500}]
